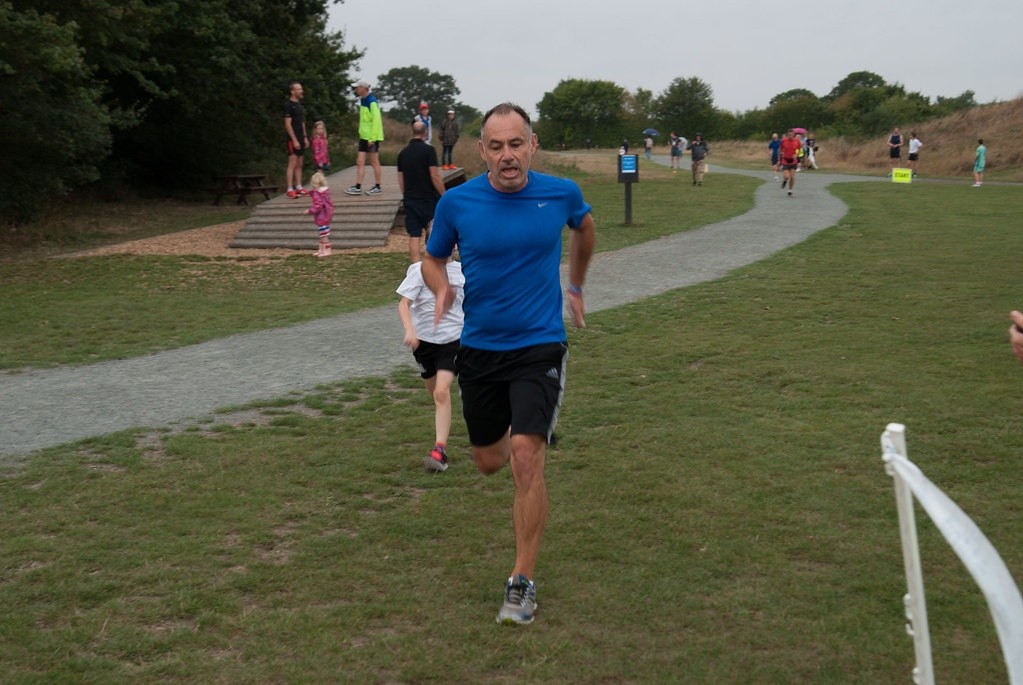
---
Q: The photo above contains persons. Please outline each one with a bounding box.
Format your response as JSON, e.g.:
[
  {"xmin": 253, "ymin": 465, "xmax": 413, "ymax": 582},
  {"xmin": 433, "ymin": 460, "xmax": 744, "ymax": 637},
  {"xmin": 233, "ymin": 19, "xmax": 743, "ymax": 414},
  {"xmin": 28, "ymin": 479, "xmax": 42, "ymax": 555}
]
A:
[
  {"xmin": 972, "ymin": 139, "xmax": 987, "ymax": 187},
  {"xmin": 343, "ymin": 80, "xmax": 384, "ymax": 195},
  {"xmin": 775, "ymin": 128, "xmax": 820, "ymax": 172},
  {"xmin": 305, "ymin": 173, "xmax": 333, "ymax": 258},
  {"xmin": 887, "ymin": 126, "xmax": 904, "ymax": 177},
  {"xmin": 311, "ymin": 120, "xmax": 331, "ymax": 176},
  {"xmin": 768, "ymin": 133, "xmax": 785, "ymax": 182},
  {"xmin": 906, "ymin": 132, "xmax": 923, "ymax": 177},
  {"xmin": 396, "ymin": 103, "xmax": 597, "ymax": 625},
  {"xmin": 397, "ymin": 122, "xmax": 447, "ymax": 271},
  {"xmin": 686, "ymin": 132, "xmax": 709, "ymax": 186},
  {"xmin": 668, "ymin": 132, "xmax": 687, "ymax": 171},
  {"xmin": 282, "ymin": 82, "xmax": 310, "ymax": 199},
  {"xmin": 1009, "ymin": 310, "xmax": 1023, "ymax": 363},
  {"xmin": 622, "ymin": 138, "xmax": 631, "ymax": 155},
  {"xmin": 644, "ymin": 136, "xmax": 653, "ymax": 160},
  {"xmin": 778, "ymin": 129, "xmax": 802, "ymax": 196}
]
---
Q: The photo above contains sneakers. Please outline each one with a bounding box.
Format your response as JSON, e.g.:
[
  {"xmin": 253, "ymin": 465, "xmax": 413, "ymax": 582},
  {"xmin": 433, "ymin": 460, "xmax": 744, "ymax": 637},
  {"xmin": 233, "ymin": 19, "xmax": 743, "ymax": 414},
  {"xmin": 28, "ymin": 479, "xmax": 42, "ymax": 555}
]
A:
[
  {"xmin": 286, "ymin": 190, "xmax": 298, "ymax": 199},
  {"xmin": 424, "ymin": 446, "xmax": 448, "ymax": 472},
  {"xmin": 296, "ymin": 189, "xmax": 312, "ymax": 197},
  {"xmin": 365, "ymin": 187, "xmax": 382, "ymax": 195},
  {"xmin": 345, "ymin": 186, "xmax": 362, "ymax": 194},
  {"xmin": 496, "ymin": 575, "xmax": 537, "ymax": 624}
]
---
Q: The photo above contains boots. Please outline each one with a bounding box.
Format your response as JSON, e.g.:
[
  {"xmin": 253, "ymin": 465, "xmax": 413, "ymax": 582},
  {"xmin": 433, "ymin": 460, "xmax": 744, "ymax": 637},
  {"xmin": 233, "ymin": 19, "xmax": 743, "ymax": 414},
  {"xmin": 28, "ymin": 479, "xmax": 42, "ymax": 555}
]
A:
[{"xmin": 313, "ymin": 243, "xmax": 332, "ymax": 258}]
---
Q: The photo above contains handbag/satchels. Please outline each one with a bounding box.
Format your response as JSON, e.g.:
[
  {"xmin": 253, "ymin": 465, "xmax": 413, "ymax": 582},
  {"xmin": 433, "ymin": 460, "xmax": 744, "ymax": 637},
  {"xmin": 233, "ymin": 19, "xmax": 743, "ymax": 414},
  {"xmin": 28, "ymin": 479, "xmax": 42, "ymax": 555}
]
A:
[{"xmin": 785, "ymin": 158, "xmax": 793, "ymax": 164}]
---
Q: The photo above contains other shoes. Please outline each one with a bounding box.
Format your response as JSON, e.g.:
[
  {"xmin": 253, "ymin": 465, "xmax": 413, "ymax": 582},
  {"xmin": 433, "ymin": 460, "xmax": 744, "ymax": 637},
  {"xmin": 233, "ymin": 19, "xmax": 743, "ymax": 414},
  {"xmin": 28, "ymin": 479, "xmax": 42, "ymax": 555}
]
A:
[
  {"xmin": 443, "ymin": 164, "xmax": 449, "ymax": 170},
  {"xmin": 450, "ymin": 164, "xmax": 456, "ymax": 169},
  {"xmin": 782, "ymin": 181, "xmax": 787, "ymax": 189},
  {"xmin": 694, "ymin": 181, "xmax": 696, "ymax": 185},
  {"xmin": 972, "ymin": 183, "xmax": 981, "ymax": 187},
  {"xmin": 796, "ymin": 169, "xmax": 800, "ymax": 172},
  {"xmin": 698, "ymin": 181, "xmax": 701, "ymax": 186},
  {"xmin": 788, "ymin": 191, "xmax": 792, "ymax": 196},
  {"xmin": 774, "ymin": 177, "xmax": 778, "ymax": 180}
]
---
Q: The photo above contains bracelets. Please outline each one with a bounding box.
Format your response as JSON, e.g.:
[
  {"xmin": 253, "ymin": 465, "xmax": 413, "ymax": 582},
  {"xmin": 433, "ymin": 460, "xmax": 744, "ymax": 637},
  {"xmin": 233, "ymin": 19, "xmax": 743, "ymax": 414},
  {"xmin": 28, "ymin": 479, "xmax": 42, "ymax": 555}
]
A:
[
  {"xmin": 567, "ymin": 284, "xmax": 584, "ymax": 299},
  {"xmin": 303, "ymin": 135, "xmax": 308, "ymax": 138}
]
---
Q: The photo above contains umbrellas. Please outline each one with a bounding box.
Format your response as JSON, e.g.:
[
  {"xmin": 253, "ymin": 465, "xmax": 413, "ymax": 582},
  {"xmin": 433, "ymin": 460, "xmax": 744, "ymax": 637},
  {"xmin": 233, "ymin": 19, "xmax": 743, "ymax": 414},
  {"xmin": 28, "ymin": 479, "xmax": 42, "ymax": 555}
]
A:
[{"xmin": 642, "ymin": 128, "xmax": 661, "ymax": 137}]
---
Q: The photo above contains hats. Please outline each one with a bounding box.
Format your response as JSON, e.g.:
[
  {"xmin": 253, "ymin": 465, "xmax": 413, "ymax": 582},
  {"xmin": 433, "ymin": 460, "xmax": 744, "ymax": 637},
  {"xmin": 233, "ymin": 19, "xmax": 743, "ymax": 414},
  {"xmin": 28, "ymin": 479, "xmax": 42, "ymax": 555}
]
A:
[
  {"xmin": 448, "ymin": 110, "xmax": 455, "ymax": 113},
  {"xmin": 420, "ymin": 104, "xmax": 428, "ymax": 109},
  {"xmin": 350, "ymin": 80, "xmax": 371, "ymax": 90}
]
